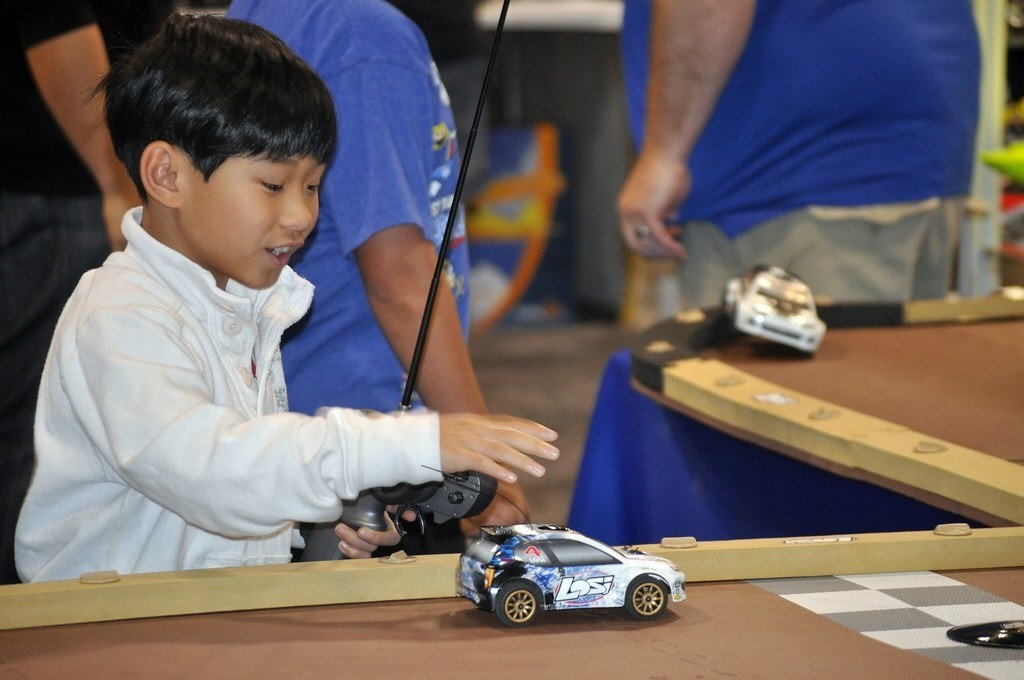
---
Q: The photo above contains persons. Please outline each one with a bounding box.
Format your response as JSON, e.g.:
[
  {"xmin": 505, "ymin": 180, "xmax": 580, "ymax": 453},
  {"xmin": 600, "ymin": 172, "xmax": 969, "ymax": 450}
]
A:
[
  {"xmin": 0, "ymin": 0, "xmax": 173, "ymax": 586},
  {"xmin": 223, "ymin": 1, "xmax": 531, "ymax": 566},
  {"xmin": 12, "ymin": 11, "xmax": 561, "ymax": 586},
  {"xmin": 618, "ymin": 0, "xmax": 985, "ymax": 310}
]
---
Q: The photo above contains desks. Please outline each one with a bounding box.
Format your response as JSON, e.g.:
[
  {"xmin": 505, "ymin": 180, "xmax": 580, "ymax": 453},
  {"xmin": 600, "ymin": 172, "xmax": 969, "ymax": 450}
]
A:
[{"xmin": 0, "ymin": 277, "xmax": 1024, "ymax": 680}]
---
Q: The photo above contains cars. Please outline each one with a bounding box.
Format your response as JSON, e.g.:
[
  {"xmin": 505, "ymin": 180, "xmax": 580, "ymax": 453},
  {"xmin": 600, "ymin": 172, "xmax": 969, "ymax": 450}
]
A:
[
  {"xmin": 719, "ymin": 265, "xmax": 826, "ymax": 359},
  {"xmin": 455, "ymin": 522, "xmax": 687, "ymax": 629}
]
